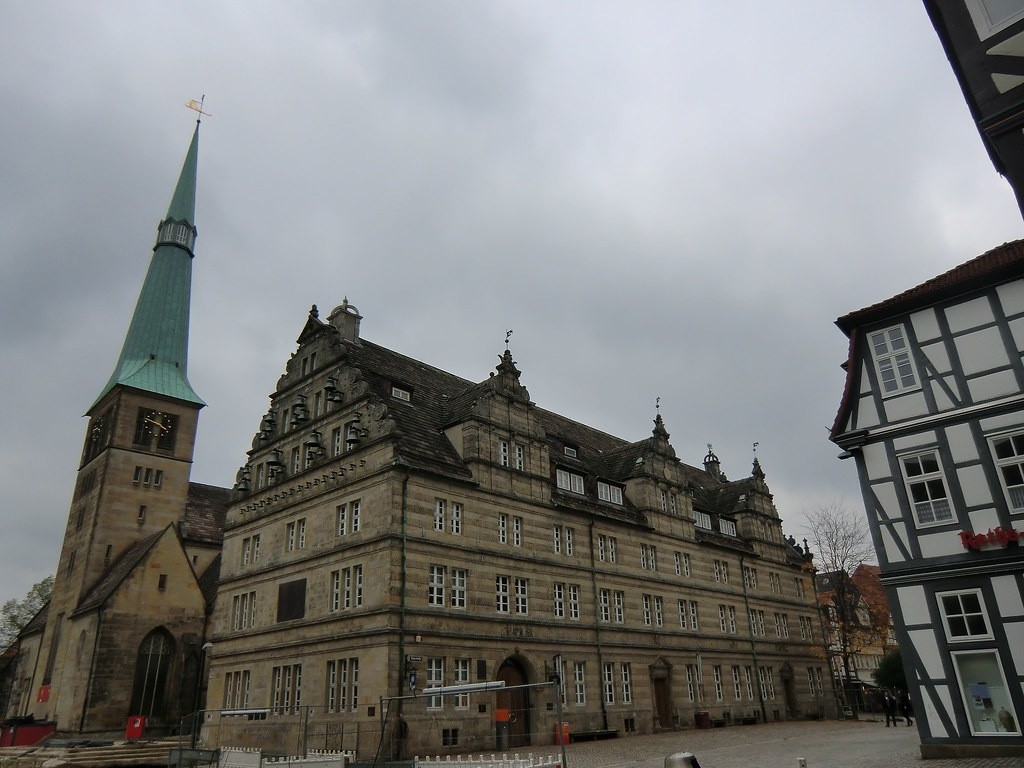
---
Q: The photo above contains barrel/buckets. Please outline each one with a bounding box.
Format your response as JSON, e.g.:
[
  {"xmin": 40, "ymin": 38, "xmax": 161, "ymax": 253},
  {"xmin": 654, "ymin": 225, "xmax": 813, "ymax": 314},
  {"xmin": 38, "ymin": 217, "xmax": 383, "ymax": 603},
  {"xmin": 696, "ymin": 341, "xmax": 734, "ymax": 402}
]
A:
[
  {"xmin": 555, "ymin": 722, "xmax": 570, "ymax": 745},
  {"xmin": 698, "ymin": 711, "xmax": 710, "ymax": 729},
  {"xmin": 496, "ymin": 720, "xmax": 516, "ymax": 752}
]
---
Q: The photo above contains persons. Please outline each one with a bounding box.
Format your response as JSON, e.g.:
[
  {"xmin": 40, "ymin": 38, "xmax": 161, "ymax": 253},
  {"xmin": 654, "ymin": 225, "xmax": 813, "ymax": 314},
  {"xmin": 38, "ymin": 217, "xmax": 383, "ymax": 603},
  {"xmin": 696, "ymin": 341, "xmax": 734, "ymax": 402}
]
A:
[
  {"xmin": 882, "ymin": 691, "xmax": 898, "ymax": 728},
  {"xmin": 896, "ymin": 689, "xmax": 913, "ymax": 727}
]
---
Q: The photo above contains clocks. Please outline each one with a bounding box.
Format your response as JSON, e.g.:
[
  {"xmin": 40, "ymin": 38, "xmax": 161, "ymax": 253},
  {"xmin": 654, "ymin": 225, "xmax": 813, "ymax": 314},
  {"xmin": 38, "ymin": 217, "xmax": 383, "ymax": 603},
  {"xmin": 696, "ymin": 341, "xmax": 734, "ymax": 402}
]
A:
[
  {"xmin": 91, "ymin": 416, "xmax": 103, "ymax": 441},
  {"xmin": 145, "ymin": 412, "xmax": 171, "ymax": 436}
]
